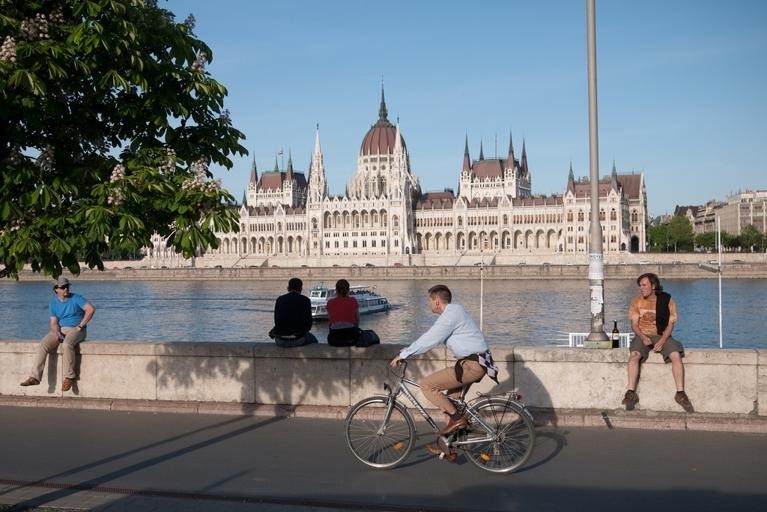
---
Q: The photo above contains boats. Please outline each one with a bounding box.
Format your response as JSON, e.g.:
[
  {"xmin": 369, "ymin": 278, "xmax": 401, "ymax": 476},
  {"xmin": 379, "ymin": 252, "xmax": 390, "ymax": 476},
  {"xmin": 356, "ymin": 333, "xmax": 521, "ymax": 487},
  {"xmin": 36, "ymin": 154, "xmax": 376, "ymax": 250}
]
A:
[{"xmin": 308, "ymin": 281, "xmax": 392, "ymax": 322}]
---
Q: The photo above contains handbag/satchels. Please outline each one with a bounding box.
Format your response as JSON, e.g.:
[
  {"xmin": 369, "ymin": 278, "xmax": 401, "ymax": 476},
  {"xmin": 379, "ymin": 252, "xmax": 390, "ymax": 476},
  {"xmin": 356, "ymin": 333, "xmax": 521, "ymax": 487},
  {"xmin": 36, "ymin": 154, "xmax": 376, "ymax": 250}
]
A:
[{"xmin": 354, "ymin": 325, "xmax": 381, "ymax": 348}]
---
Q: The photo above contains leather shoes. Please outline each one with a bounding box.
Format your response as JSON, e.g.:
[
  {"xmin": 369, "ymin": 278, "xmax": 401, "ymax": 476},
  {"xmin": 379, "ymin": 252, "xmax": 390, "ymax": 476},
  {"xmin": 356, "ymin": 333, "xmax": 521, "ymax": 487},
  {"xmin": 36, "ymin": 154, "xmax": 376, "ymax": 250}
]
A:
[
  {"xmin": 424, "ymin": 440, "xmax": 460, "ymax": 464},
  {"xmin": 436, "ymin": 418, "xmax": 470, "ymax": 436}
]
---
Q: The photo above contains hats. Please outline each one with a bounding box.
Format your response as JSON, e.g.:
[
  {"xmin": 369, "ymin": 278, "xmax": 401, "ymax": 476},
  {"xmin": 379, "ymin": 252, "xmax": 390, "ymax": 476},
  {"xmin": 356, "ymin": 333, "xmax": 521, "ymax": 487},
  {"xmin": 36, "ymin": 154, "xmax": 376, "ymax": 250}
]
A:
[{"xmin": 51, "ymin": 276, "xmax": 75, "ymax": 290}]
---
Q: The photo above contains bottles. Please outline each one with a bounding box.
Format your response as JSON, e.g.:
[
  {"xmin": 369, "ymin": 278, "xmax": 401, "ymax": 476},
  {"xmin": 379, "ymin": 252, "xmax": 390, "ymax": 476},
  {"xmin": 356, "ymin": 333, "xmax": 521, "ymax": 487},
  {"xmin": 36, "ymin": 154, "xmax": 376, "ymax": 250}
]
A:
[{"xmin": 611, "ymin": 320, "xmax": 619, "ymax": 348}]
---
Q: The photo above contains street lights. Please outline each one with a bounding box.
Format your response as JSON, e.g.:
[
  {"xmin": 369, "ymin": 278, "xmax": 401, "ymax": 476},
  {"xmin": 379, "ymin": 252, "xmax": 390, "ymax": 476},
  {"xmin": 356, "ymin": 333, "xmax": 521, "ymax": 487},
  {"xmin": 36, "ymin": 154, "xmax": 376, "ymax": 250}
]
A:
[{"xmin": 698, "ymin": 263, "xmax": 722, "ymax": 349}]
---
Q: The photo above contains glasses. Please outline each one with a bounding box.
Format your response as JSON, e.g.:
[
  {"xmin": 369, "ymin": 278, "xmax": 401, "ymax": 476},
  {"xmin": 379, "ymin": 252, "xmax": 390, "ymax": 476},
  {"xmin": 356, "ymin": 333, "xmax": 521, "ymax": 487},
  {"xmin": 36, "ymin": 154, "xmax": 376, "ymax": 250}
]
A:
[{"xmin": 58, "ymin": 284, "xmax": 70, "ymax": 290}]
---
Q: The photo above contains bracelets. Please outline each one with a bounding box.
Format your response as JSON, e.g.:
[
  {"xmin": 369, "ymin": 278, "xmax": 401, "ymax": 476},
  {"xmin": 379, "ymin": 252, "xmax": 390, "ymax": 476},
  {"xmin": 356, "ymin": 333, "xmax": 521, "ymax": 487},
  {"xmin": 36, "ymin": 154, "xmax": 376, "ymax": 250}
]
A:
[{"xmin": 77, "ymin": 325, "xmax": 82, "ymax": 329}]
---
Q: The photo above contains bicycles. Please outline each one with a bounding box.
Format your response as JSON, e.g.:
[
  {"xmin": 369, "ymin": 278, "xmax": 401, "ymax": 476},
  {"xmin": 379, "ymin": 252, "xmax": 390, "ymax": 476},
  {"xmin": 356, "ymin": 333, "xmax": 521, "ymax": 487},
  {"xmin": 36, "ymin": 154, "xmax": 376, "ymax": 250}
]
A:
[{"xmin": 343, "ymin": 360, "xmax": 539, "ymax": 475}]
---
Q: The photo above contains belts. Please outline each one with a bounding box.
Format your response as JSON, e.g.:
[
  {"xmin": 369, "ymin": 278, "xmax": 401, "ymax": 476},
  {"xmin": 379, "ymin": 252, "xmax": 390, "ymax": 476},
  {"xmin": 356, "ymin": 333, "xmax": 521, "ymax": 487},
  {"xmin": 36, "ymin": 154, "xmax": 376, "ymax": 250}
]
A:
[{"xmin": 275, "ymin": 335, "xmax": 306, "ymax": 341}]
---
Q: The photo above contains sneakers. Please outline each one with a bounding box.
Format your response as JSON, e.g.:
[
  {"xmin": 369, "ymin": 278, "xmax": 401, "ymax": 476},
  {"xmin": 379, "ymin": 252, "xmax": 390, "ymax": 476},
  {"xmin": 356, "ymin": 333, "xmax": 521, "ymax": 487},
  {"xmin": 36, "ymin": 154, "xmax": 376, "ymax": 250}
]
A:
[
  {"xmin": 673, "ymin": 391, "xmax": 695, "ymax": 412},
  {"xmin": 60, "ymin": 375, "xmax": 77, "ymax": 393},
  {"xmin": 620, "ymin": 390, "xmax": 643, "ymax": 408},
  {"xmin": 17, "ymin": 377, "xmax": 43, "ymax": 388}
]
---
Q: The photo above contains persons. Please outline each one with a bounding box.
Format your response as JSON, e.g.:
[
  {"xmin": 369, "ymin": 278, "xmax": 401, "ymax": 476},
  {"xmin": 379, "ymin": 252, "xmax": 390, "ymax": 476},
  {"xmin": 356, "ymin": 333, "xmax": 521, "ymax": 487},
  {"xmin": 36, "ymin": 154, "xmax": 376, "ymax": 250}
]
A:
[
  {"xmin": 325, "ymin": 279, "xmax": 380, "ymax": 347},
  {"xmin": 19, "ymin": 276, "xmax": 95, "ymax": 392},
  {"xmin": 619, "ymin": 272, "xmax": 694, "ymax": 411},
  {"xmin": 267, "ymin": 277, "xmax": 319, "ymax": 348},
  {"xmin": 389, "ymin": 284, "xmax": 500, "ymax": 464}
]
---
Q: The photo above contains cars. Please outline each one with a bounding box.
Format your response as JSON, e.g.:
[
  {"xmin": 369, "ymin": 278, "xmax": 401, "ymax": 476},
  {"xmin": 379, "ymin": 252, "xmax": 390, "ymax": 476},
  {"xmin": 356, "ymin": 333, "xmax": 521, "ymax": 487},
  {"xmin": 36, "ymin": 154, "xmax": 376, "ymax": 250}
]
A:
[{"xmin": 617, "ymin": 259, "xmax": 744, "ymax": 265}]
